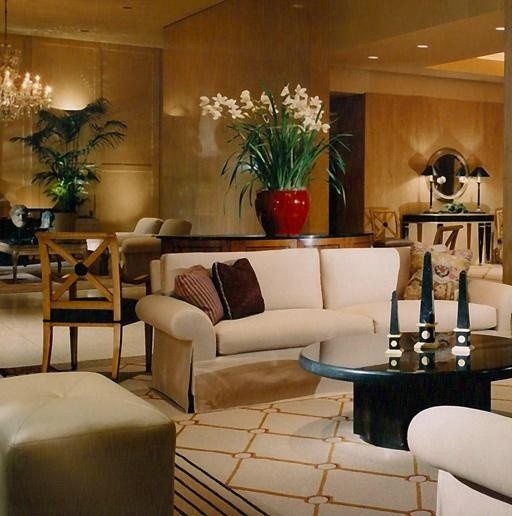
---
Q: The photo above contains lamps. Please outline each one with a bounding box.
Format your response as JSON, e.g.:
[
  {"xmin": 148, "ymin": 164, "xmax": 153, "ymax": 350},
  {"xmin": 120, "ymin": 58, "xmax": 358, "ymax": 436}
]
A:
[
  {"xmin": 468, "ymin": 167, "xmax": 490, "ymax": 213},
  {"xmin": 454, "ymin": 167, "xmax": 465, "ymax": 183},
  {"xmin": 421, "ymin": 165, "xmax": 439, "ymax": 214},
  {"xmin": 0, "ymin": 0, "xmax": 54, "ymax": 126}
]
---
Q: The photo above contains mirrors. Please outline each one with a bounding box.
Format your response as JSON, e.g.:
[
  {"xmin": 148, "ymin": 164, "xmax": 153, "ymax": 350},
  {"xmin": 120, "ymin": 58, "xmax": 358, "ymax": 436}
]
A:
[{"xmin": 425, "ymin": 147, "xmax": 469, "ymax": 201}]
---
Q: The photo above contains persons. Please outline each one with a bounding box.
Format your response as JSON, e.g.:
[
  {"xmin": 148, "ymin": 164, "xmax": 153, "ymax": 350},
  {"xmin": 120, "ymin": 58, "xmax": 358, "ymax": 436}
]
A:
[{"xmin": 0, "ymin": 204, "xmax": 36, "ymax": 245}]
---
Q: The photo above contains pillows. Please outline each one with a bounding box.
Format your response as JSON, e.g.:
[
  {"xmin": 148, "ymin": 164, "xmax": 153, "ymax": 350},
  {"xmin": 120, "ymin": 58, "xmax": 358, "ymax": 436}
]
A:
[
  {"xmin": 398, "ymin": 243, "xmax": 473, "ymax": 300},
  {"xmin": 212, "ymin": 258, "xmax": 265, "ymax": 319},
  {"xmin": 175, "ymin": 264, "xmax": 224, "ymax": 324}
]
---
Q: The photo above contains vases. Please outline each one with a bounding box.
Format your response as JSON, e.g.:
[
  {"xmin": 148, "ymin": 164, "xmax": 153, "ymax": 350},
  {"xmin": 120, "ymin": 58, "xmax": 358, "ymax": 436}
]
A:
[{"xmin": 255, "ymin": 190, "xmax": 312, "ymax": 239}]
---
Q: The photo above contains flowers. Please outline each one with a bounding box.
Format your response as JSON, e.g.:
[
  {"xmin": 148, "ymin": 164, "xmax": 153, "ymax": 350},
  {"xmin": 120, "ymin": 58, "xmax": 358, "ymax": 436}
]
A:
[{"xmin": 199, "ymin": 80, "xmax": 354, "ymax": 220}]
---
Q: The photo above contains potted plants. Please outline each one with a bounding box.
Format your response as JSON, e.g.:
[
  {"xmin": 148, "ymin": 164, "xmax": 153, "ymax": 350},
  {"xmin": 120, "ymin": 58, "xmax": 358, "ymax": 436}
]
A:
[{"xmin": 9, "ymin": 98, "xmax": 127, "ymax": 243}]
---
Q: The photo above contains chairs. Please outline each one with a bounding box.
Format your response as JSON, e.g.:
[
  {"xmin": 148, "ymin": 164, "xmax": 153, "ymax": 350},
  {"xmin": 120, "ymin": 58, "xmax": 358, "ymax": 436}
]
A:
[
  {"xmin": 371, "ymin": 210, "xmax": 414, "ymax": 247},
  {"xmin": 35, "ymin": 232, "xmax": 153, "ymax": 381},
  {"xmin": 118, "ymin": 218, "xmax": 192, "ymax": 283},
  {"xmin": 433, "ymin": 225, "xmax": 464, "ymax": 250},
  {"xmin": 108, "ymin": 217, "xmax": 164, "ymax": 279}
]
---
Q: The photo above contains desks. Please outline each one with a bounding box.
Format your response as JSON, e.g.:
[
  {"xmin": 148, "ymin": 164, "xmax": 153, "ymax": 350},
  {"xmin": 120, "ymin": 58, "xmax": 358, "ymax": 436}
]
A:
[
  {"xmin": 400, "ymin": 212, "xmax": 498, "ymax": 266},
  {"xmin": 156, "ymin": 233, "xmax": 374, "ymax": 251},
  {"xmin": 0, "ymin": 239, "xmax": 92, "ymax": 285}
]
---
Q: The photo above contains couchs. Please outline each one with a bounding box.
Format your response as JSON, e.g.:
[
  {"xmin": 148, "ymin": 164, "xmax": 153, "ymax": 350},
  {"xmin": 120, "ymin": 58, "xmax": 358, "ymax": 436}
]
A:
[
  {"xmin": 134, "ymin": 246, "xmax": 512, "ymax": 412},
  {"xmin": 407, "ymin": 405, "xmax": 512, "ymax": 516}
]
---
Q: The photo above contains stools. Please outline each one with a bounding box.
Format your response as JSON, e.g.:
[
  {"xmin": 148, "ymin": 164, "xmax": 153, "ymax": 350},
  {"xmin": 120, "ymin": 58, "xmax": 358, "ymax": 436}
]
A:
[{"xmin": 1, "ymin": 371, "xmax": 176, "ymax": 516}]
